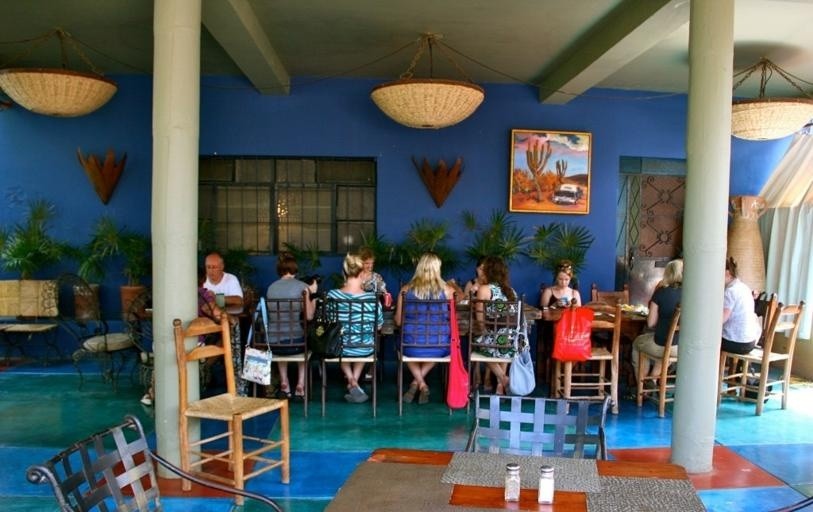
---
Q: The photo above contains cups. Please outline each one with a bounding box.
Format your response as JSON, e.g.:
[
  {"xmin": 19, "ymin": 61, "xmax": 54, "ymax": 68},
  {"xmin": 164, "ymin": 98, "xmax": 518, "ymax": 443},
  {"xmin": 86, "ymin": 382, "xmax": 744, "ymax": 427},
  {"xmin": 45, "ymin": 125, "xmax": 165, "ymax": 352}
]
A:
[
  {"xmin": 216, "ymin": 293, "xmax": 224, "ymax": 307},
  {"xmin": 384, "ymin": 293, "xmax": 391, "ymax": 307}
]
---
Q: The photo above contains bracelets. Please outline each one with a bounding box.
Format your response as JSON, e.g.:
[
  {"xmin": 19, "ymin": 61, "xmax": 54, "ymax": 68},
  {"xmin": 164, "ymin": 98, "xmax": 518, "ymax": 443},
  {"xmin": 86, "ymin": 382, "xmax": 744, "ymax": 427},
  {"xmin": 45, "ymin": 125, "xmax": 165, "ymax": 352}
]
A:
[{"xmin": 311, "ymin": 291, "xmax": 318, "ymax": 301}]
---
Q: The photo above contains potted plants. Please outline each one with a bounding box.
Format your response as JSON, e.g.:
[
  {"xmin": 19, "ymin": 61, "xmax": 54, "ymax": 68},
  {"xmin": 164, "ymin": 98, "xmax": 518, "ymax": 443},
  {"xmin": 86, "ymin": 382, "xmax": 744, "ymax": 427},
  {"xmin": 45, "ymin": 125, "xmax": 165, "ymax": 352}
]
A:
[
  {"xmin": 119, "ymin": 236, "xmax": 148, "ymax": 324},
  {"xmin": 63, "ymin": 214, "xmax": 120, "ymax": 323}
]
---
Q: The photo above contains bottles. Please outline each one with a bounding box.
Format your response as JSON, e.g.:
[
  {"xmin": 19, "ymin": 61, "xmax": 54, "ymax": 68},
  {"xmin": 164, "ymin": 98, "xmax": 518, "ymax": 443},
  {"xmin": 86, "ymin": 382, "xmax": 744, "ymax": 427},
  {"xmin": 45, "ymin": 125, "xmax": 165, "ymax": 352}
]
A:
[
  {"xmin": 504, "ymin": 463, "xmax": 521, "ymax": 502},
  {"xmin": 538, "ymin": 465, "xmax": 555, "ymax": 504}
]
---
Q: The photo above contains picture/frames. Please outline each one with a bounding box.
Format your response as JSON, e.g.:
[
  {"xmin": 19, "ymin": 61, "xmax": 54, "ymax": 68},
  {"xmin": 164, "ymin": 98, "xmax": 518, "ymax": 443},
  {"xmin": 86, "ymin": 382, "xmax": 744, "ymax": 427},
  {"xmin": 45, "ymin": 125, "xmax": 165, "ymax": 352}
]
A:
[{"xmin": 509, "ymin": 129, "xmax": 592, "ymax": 214}]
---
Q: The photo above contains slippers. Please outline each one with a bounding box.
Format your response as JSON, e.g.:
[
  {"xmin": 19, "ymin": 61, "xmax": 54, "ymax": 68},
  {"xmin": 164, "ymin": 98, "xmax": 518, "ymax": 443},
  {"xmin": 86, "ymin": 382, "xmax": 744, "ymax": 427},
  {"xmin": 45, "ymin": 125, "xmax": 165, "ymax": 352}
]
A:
[
  {"xmin": 402, "ymin": 384, "xmax": 417, "ymax": 403},
  {"xmin": 419, "ymin": 386, "xmax": 430, "ymax": 404},
  {"xmin": 344, "ymin": 386, "xmax": 369, "ymax": 403},
  {"xmin": 276, "ymin": 391, "xmax": 305, "ymax": 403}
]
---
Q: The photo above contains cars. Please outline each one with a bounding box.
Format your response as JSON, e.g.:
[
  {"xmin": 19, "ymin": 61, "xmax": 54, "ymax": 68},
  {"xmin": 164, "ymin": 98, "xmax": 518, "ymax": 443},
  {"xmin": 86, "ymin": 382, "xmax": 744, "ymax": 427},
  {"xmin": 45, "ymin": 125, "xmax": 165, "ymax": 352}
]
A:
[{"xmin": 551, "ymin": 184, "xmax": 582, "ymax": 205}]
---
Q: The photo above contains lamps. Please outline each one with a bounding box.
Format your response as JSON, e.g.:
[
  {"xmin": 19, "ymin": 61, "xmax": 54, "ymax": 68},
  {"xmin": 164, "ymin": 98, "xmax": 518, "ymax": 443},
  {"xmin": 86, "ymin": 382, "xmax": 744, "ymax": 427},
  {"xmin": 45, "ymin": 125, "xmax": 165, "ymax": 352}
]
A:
[
  {"xmin": 732, "ymin": 56, "xmax": 813, "ymax": 141},
  {"xmin": 368, "ymin": 34, "xmax": 486, "ymax": 130},
  {"xmin": 0, "ymin": 28, "xmax": 118, "ymax": 117}
]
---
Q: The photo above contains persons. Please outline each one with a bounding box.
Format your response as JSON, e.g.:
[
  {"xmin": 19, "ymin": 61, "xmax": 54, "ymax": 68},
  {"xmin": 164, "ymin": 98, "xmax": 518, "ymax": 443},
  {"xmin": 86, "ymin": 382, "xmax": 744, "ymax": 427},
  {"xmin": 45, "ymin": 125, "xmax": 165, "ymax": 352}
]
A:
[
  {"xmin": 322, "ymin": 250, "xmax": 384, "ymax": 404},
  {"xmin": 473, "ymin": 254, "xmax": 522, "ymax": 404},
  {"xmin": 540, "ymin": 257, "xmax": 582, "ymax": 386},
  {"xmin": 627, "ymin": 258, "xmax": 685, "ymax": 403},
  {"xmin": 720, "ymin": 256, "xmax": 763, "ymax": 404},
  {"xmin": 203, "ymin": 254, "xmax": 247, "ymax": 370},
  {"xmin": 392, "ymin": 251, "xmax": 459, "ymax": 406},
  {"xmin": 343, "ymin": 245, "xmax": 388, "ymax": 380},
  {"xmin": 198, "ymin": 263, "xmax": 235, "ymax": 397},
  {"xmin": 462, "ymin": 254, "xmax": 493, "ymax": 392},
  {"xmin": 266, "ymin": 251, "xmax": 318, "ymax": 403}
]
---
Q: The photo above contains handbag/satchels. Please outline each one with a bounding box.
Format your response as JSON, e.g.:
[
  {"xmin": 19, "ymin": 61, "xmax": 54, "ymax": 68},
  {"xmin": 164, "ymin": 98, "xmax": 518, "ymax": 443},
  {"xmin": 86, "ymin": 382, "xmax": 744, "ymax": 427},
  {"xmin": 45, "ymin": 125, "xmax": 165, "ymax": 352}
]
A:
[
  {"xmin": 241, "ymin": 347, "xmax": 272, "ymax": 386},
  {"xmin": 308, "ymin": 320, "xmax": 343, "ymax": 356},
  {"xmin": 754, "ymin": 292, "xmax": 768, "ymax": 317},
  {"xmin": 446, "ymin": 342, "xmax": 471, "ymax": 409},
  {"xmin": 509, "ymin": 346, "xmax": 536, "ymax": 396},
  {"xmin": 551, "ymin": 307, "xmax": 594, "ymax": 362}
]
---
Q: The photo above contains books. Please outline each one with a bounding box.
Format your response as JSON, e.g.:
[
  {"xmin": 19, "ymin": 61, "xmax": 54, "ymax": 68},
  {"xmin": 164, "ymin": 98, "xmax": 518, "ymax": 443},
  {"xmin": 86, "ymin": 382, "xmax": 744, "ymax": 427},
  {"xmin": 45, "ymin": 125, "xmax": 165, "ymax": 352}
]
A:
[{"xmin": 446, "ymin": 278, "xmax": 465, "ymax": 305}]
